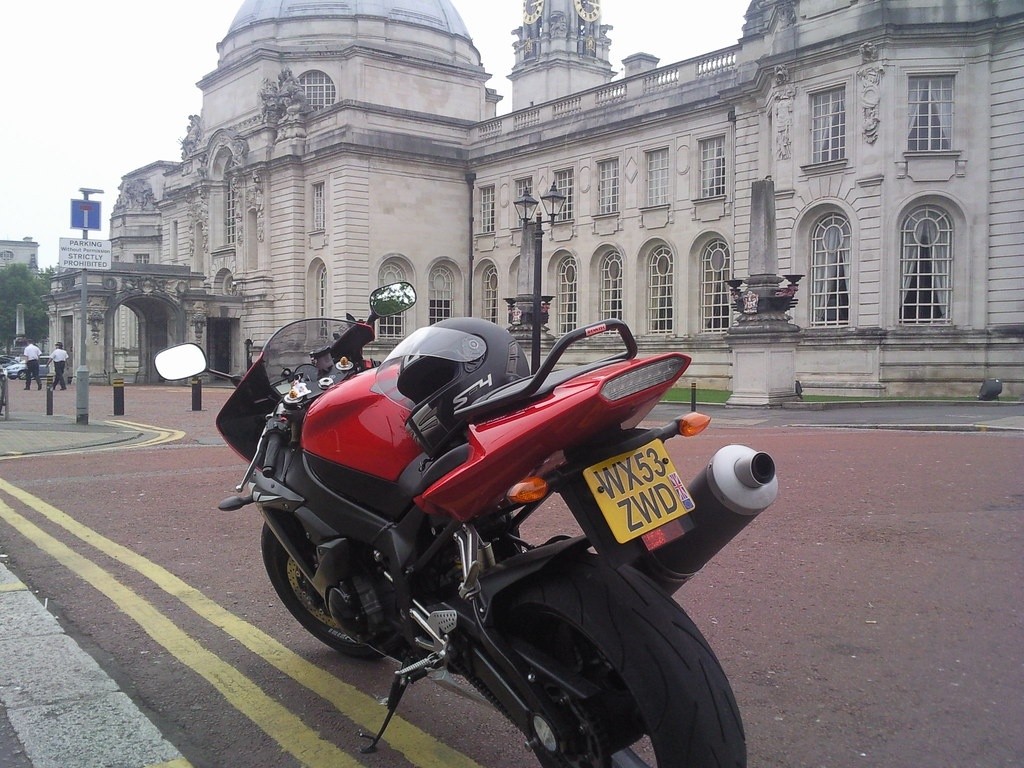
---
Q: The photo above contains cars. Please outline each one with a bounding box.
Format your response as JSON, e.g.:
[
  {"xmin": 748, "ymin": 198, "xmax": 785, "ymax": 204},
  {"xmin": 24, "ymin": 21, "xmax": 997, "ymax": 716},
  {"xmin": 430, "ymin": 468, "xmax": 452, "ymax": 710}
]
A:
[{"xmin": 6, "ymin": 355, "xmax": 51, "ymax": 379}]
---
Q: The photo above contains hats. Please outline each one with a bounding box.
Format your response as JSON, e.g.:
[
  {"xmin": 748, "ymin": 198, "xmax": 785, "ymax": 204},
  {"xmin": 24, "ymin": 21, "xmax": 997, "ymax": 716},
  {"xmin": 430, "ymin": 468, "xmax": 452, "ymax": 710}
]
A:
[{"xmin": 55, "ymin": 342, "xmax": 62, "ymax": 347}]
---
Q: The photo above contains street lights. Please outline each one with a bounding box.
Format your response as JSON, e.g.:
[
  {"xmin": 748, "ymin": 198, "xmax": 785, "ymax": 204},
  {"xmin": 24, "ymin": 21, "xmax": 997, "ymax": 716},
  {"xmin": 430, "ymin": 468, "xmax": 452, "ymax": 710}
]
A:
[{"xmin": 513, "ymin": 181, "xmax": 567, "ymax": 374}]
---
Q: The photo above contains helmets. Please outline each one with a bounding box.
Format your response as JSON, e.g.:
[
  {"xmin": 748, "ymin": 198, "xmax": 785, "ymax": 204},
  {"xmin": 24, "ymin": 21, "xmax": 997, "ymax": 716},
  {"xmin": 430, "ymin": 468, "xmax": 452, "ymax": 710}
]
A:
[{"xmin": 396, "ymin": 316, "xmax": 530, "ymax": 458}]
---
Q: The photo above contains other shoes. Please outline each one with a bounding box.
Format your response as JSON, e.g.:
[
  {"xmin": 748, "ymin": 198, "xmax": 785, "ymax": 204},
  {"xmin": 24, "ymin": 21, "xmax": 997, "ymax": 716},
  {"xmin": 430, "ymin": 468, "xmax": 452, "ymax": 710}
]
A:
[
  {"xmin": 24, "ymin": 388, "xmax": 30, "ymax": 390},
  {"xmin": 38, "ymin": 383, "xmax": 42, "ymax": 390},
  {"xmin": 60, "ymin": 387, "xmax": 66, "ymax": 390}
]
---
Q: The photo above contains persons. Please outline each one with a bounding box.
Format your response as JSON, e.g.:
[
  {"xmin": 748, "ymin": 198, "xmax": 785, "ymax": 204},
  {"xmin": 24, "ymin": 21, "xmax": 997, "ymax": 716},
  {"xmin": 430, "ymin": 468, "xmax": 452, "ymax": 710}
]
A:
[
  {"xmin": 277, "ymin": 68, "xmax": 306, "ymax": 121},
  {"xmin": 46, "ymin": 342, "xmax": 69, "ymax": 390},
  {"xmin": 184, "ymin": 115, "xmax": 198, "ymax": 143},
  {"xmin": 111, "ymin": 180, "xmax": 133, "ymax": 214},
  {"xmin": 196, "ymin": 154, "xmax": 207, "ymax": 177},
  {"xmin": 24, "ymin": 339, "xmax": 42, "ymax": 390},
  {"xmin": 229, "ymin": 140, "xmax": 244, "ymax": 167}
]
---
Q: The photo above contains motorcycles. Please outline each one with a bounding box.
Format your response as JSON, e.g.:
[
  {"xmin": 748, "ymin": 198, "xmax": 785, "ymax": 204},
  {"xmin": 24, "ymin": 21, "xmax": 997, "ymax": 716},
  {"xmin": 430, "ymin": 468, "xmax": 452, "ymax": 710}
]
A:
[{"xmin": 156, "ymin": 282, "xmax": 782, "ymax": 768}]
